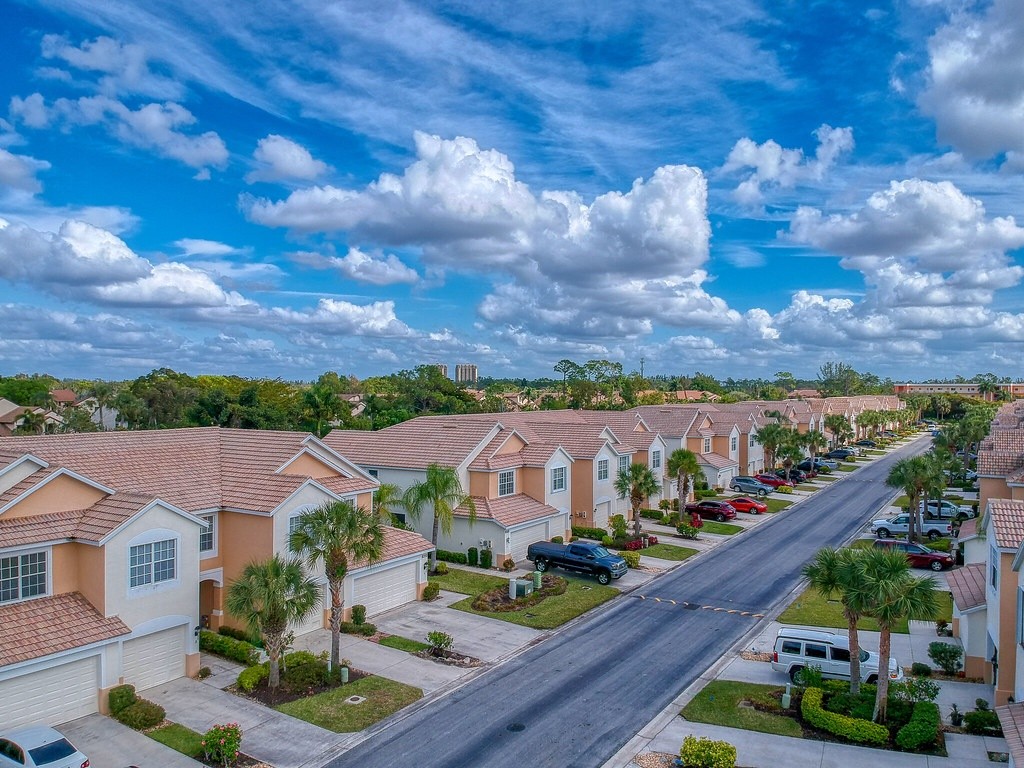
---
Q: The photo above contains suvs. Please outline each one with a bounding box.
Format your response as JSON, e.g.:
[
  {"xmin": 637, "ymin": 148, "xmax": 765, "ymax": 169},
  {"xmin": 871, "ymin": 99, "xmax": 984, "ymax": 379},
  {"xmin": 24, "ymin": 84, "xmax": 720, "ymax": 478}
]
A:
[
  {"xmin": 772, "ymin": 468, "xmax": 807, "ymax": 484},
  {"xmin": 932, "ymin": 429, "xmax": 941, "ymax": 437},
  {"xmin": 824, "ymin": 449, "xmax": 851, "ymax": 459},
  {"xmin": 771, "ymin": 626, "xmax": 904, "ymax": 688},
  {"xmin": 918, "ymin": 499, "xmax": 976, "ymax": 521},
  {"xmin": 956, "ymin": 451, "xmax": 978, "ymax": 460},
  {"xmin": 728, "ymin": 475, "xmax": 774, "ymax": 496},
  {"xmin": 683, "ymin": 499, "xmax": 737, "ymax": 523},
  {"xmin": 871, "ymin": 538, "xmax": 955, "ymax": 571}
]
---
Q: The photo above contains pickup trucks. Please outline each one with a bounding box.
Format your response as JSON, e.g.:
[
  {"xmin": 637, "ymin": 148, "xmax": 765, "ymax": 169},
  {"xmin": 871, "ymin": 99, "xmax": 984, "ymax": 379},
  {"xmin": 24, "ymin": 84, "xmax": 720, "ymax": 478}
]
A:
[
  {"xmin": 871, "ymin": 512, "xmax": 953, "ymax": 541},
  {"xmin": 525, "ymin": 538, "xmax": 629, "ymax": 586},
  {"xmin": 800, "ymin": 456, "xmax": 839, "ymax": 471}
]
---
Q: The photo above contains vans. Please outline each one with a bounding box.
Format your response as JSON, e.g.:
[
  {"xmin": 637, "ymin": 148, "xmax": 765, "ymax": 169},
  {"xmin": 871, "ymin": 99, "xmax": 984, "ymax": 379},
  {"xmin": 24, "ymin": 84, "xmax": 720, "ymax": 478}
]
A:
[{"xmin": 928, "ymin": 425, "xmax": 936, "ymax": 431}]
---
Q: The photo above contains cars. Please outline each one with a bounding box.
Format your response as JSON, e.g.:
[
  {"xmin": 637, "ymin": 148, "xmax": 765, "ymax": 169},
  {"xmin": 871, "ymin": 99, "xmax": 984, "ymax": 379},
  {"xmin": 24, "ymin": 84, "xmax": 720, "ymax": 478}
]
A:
[
  {"xmin": 754, "ymin": 473, "xmax": 794, "ymax": 489},
  {"xmin": 876, "ymin": 431, "xmax": 897, "ymax": 438},
  {"xmin": 727, "ymin": 496, "xmax": 768, "ymax": 514},
  {"xmin": 842, "ymin": 446, "xmax": 859, "ymax": 454},
  {"xmin": 0, "ymin": 722, "xmax": 92, "ymax": 768},
  {"xmin": 792, "ymin": 463, "xmax": 830, "ymax": 474},
  {"xmin": 855, "ymin": 440, "xmax": 876, "ymax": 448}
]
